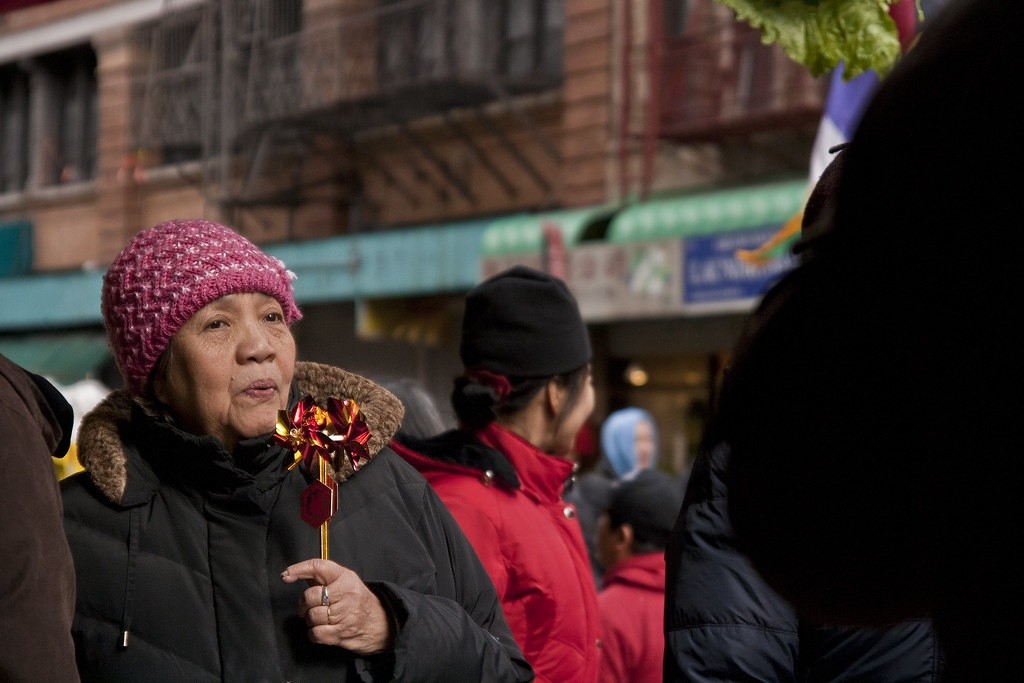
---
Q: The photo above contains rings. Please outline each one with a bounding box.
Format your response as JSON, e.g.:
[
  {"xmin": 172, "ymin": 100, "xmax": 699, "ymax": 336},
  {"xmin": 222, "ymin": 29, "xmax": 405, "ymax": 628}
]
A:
[
  {"xmin": 328, "ymin": 607, "xmax": 330, "ymax": 624},
  {"xmin": 322, "ymin": 584, "xmax": 329, "ymax": 606}
]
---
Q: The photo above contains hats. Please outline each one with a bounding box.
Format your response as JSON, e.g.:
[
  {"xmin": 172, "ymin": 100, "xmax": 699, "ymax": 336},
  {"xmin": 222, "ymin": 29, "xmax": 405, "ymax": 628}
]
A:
[
  {"xmin": 581, "ymin": 467, "xmax": 691, "ymax": 536},
  {"xmin": 461, "ymin": 264, "xmax": 592, "ymax": 378},
  {"xmin": 100, "ymin": 218, "xmax": 303, "ymax": 396},
  {"xmin": 43, "ymin": 375, "xmax": 112, "ymax": 443}
]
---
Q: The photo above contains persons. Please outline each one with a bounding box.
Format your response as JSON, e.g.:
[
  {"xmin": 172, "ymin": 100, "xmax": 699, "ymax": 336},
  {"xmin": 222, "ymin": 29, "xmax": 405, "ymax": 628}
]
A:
[
  {"xmin": 593, "ymin": 464, "xmax": 677, "ymax": 682},
  {"xmin": 36, "ymin": 346, "xmax": 710, "ymax": 590},
  {"xmin": 51, "ymin": 215, "xmax": 539, "ymax": 683},
  {"xmin": 0, "ymin": 354, "xmax": 82, "ymax": 682},
  {"xmin": 656, "ymin": 0, "xmax": 1024, "ymax": 682},
  {"xmin": 387, "ymin": 265, "xmax": 628, "ymax": 683}
]
